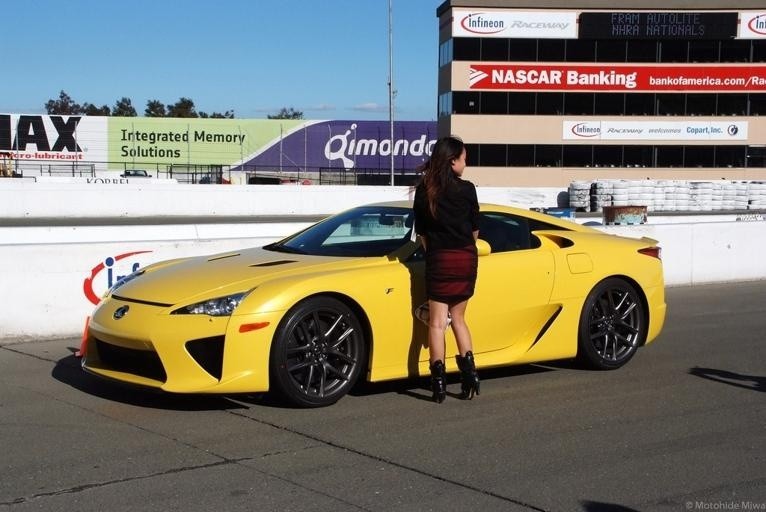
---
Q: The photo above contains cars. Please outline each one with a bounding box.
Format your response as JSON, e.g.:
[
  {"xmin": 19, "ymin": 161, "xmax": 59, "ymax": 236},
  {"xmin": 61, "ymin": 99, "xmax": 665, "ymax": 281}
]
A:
[
  {"xmin": 199, "ymin": 177, "xmax": 229, "ymax": 184},
  {"xmin": 81, "ymin": 200, "xmax": 667, "ymax": 408}
]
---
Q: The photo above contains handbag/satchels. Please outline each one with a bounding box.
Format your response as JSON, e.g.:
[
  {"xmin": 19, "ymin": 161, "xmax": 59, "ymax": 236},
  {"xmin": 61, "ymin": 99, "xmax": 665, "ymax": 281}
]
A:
[{"xmin": 415, "ymin": 300, "xmax": 451, "ymax": 331}]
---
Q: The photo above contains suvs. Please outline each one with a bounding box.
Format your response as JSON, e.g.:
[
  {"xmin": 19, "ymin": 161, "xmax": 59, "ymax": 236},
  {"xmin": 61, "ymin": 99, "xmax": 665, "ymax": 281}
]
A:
[{"xmin": 120, "ymin": 170, "xmax": 152, "ymax": 177}]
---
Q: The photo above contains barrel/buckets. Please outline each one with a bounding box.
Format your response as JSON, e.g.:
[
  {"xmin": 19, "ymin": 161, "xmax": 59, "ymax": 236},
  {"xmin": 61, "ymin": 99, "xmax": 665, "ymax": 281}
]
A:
[
  {"xmin": 546, "ymin": 207, "xmax": 577, "ymax": 219},
  {"xmin": 603, "ymin": 205, "xmax": 648, "ymax": 224}
]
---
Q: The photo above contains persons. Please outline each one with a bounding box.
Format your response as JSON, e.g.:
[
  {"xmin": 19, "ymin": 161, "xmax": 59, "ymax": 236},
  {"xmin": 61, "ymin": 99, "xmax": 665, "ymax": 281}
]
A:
[{"xmin": 415, "ymin": 134, "xmax": 481, "ymax": 403}]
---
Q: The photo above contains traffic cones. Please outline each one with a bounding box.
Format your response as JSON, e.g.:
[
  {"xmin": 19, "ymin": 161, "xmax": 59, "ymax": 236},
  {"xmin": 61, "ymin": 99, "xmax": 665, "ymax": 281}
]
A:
[{"xmin": 75, "ymin": 317, "xmax": 90, "ymax": 357}]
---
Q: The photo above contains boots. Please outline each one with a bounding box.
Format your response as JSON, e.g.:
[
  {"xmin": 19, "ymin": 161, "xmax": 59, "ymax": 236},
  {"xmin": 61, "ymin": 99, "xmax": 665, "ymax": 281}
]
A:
[
  {"xmin": 430, "ymin": 360, "xmax": 447, "ymax": 402},
  {"xmin": 456, "ymin": 350, "xmax": 480, "ymax": 400}
]
